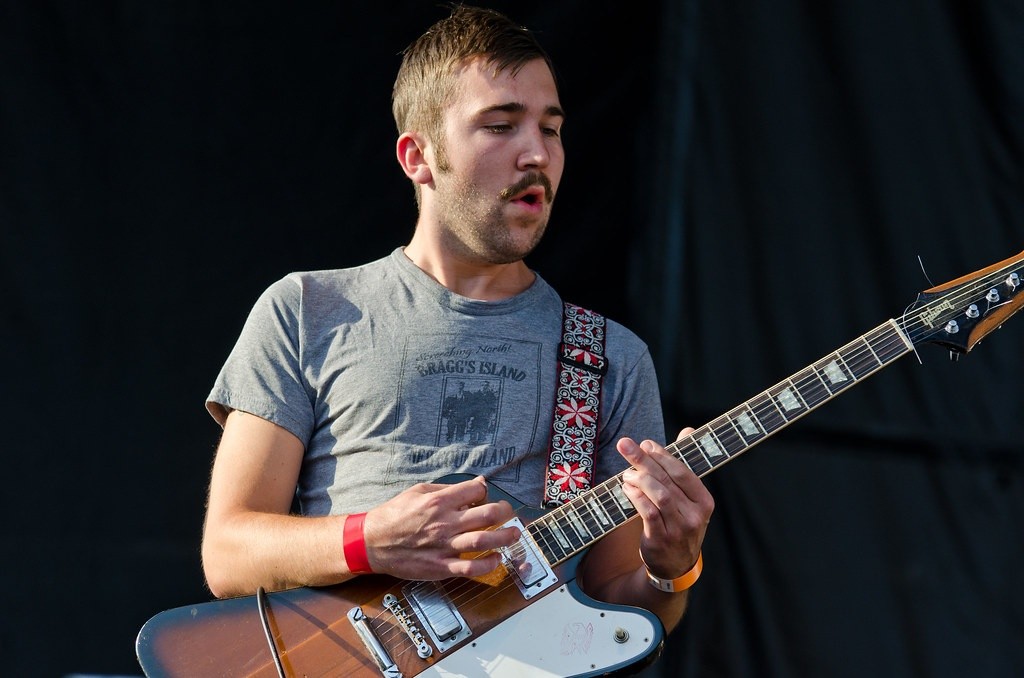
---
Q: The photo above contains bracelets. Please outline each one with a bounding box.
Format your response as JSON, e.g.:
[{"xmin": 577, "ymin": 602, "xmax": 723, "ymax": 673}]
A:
[
  {"xmin": 638, "ymin": 547, "xmax": 703, "ymax": 594},
  {"xmin": 343, "ymin": 512, "xmax": 377, "ymax": 575}
]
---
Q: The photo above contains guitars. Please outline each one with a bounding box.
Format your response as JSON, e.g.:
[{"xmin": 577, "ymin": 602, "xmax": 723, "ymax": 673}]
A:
[{"xmin": 133, "ymin": 248, "xmax": 1024, "ymax": 677}]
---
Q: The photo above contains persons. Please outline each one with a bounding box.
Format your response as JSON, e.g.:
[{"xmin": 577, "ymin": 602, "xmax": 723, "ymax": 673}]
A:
[{"xmin": 200, "ymin": 6, "xmax": 717, "ymax": 678}]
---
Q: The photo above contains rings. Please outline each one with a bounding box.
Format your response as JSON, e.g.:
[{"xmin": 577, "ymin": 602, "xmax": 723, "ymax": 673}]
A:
[{"xmin": 471, "ymin": 478, "xmax": 489, "ymax": 505}]
